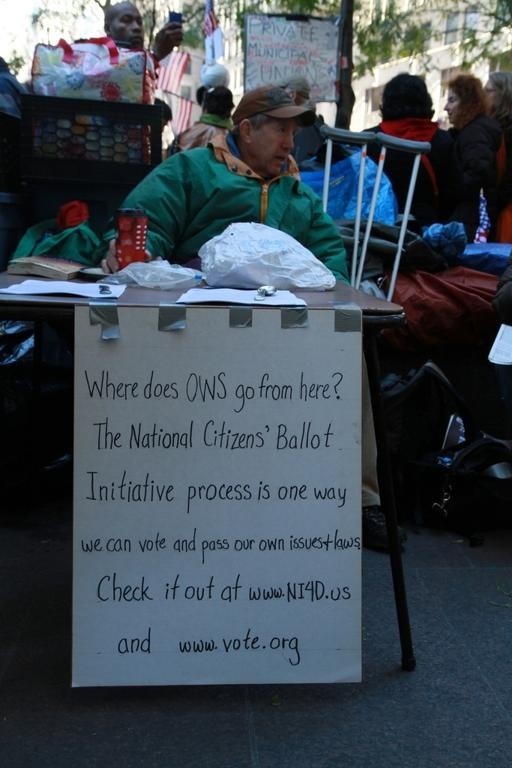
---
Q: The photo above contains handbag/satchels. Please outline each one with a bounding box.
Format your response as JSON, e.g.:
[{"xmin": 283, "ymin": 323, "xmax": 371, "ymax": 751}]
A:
[
  {"xmin": 31, "ymin": 37, "xmax": 160, "ymax": 166},
  {"xmin": 337, "ymin": 216, "xmax": 435, "ymax": 265},
  {"xmin": 13, "ymin": 215, "xmax": 101, "ymax": 264},
  {"xmin": 376, "ymin": 360, "xmax": 512, "ymax": 547}
]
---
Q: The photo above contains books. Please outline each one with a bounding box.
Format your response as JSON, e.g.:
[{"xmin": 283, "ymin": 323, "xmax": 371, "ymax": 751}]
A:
[{"xmin": 7, "ymin": 256, "xmax": 90, "ymax": 280}]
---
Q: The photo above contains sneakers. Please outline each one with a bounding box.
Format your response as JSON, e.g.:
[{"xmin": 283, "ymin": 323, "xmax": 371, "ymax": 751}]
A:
[{"xmin": 363, "ymin": 506, "xmax": 407, "ymax": 549}]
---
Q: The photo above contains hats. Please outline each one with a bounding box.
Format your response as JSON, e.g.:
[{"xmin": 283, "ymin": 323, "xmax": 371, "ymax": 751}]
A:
[
  {"xmin": 279, "ymin": 75, "xmax": 310, "ymax": 98},
  {"xmin": 1, "ymin": 93, "xmax": 20, "ymax": 119},
  {"xmin": 205, "ymin": 85, "xmax": 234, "ymax": 117},
  {"xmin": 232, "ymin": 83, "xmax": 316, "ymax": 128}
]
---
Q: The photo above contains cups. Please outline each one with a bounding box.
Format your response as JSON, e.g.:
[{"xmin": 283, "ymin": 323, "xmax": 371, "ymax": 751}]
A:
[{"xmin": 115, "ymin": 206, "xmax": 148, "ymax": 270}]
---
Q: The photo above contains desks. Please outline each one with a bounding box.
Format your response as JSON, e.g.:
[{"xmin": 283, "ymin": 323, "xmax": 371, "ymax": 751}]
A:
[{"xmin": 0, "ymin": 266, "xmax": 416, "ymax": 672}]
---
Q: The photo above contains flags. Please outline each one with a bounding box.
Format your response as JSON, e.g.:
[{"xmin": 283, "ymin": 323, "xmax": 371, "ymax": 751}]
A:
[{"xmin": 204, "ymin": 0, "xmax": 225, "ymax": 63}]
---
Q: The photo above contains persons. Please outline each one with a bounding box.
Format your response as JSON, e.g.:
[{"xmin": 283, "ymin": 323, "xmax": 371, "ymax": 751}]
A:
[{"xmin": 92, "ymin": 86, "xmax": 408, "ymax": 553}]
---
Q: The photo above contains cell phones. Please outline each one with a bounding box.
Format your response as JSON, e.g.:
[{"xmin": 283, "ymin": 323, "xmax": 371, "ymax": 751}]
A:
[{"xmin": 170, "ymin": 13, "xmax": 182, "ymax": 40}]
